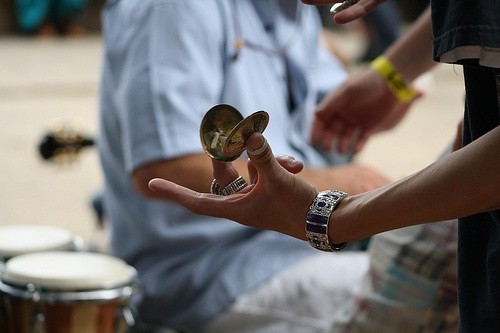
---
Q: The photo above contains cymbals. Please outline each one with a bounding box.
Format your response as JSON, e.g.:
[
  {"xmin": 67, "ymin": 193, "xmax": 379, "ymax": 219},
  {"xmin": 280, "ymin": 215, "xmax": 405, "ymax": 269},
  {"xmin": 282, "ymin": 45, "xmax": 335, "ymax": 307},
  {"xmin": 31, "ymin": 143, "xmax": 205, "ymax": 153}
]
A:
[{"xmin": 199, "ymin": 103, "xmax": 270, "ymax": 163}]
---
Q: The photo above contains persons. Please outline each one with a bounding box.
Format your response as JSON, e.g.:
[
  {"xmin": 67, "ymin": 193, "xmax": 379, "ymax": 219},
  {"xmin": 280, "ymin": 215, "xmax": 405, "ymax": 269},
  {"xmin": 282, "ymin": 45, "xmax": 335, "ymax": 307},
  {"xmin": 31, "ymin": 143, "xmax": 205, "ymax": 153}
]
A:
[
  {"xmin": 97, "ymin": 0, "xmax": 395, "ymax": 333},
  {"xmin": 148, "ymin": 1, "xmax": 500, "ymax": 333}
]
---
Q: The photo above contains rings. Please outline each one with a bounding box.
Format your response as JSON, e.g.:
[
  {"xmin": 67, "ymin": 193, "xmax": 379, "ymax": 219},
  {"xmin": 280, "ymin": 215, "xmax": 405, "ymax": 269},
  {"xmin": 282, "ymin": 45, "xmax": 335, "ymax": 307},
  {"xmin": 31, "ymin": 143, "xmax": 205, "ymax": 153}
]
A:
[{"xmin": 210, "ymin": 176, "xmax": 247, "ymax": 196}]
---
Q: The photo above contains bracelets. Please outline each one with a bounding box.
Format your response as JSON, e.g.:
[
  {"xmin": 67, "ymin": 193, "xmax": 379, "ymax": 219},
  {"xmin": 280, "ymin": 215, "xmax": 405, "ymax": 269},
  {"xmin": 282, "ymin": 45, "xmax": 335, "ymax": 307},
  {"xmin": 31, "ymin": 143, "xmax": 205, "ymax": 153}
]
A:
[
  {"xmin": 370, "ymin": 55, "xmax": 422, "ymax": 105},
  {"xmin": 305, "ymin": 189, "xmax": 351, "ymax": 252}
]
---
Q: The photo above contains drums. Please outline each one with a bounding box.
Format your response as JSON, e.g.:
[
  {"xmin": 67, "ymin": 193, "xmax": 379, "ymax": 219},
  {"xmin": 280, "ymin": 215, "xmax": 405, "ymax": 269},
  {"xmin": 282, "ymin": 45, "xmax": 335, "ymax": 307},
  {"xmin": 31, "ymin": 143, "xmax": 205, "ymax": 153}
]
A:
[
  {"xmin": 0, "ymin": 225, "xmax": 88, "ymax": 260},
  {"xmin": 0, "ymin": 249, "xmax": 137, "ymax": 333}
]
